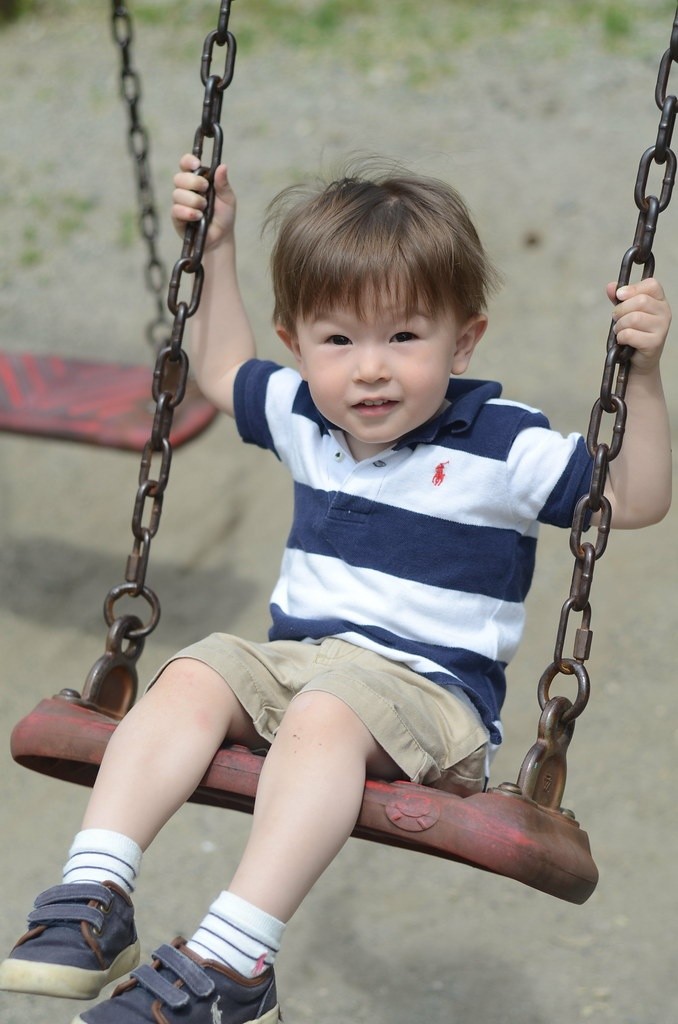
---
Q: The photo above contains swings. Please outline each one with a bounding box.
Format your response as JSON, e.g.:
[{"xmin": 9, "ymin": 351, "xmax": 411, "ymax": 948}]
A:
[
  {"xmin": 6, "ymin": 0, "xmax": 677, "ymax": 908},
  {"xmin": 0, "ymin": 0, "xmax": 218, "ymax": 455}
]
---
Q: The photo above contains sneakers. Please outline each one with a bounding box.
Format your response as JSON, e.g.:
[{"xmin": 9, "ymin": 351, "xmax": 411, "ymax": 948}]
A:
[
  {"xmin": 0, "ymin": 880, "xmax": 141, "ymax": 1000},
  {"xmin": 72, "ymin": 936, "xmax": 283, "ymax": 1024}
]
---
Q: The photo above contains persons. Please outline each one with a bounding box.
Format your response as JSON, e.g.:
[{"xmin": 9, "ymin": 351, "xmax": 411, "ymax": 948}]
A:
[{"xmin": 0, "ymin": 154, "xmax": 671, "ymax": 1024}]
